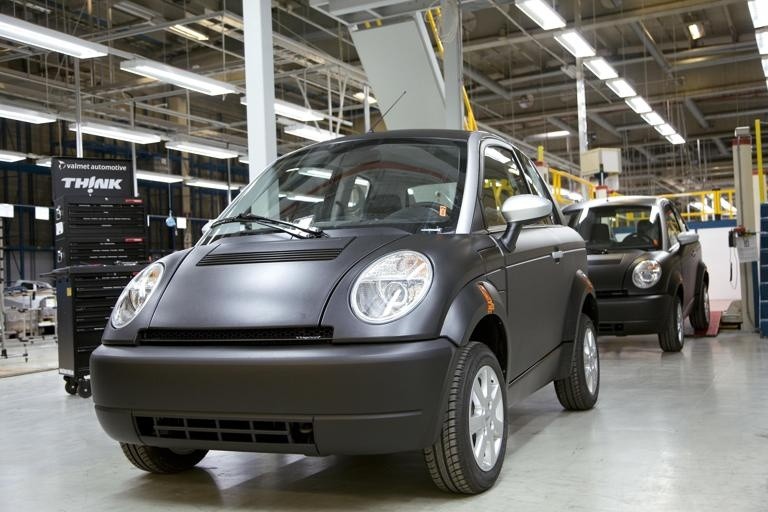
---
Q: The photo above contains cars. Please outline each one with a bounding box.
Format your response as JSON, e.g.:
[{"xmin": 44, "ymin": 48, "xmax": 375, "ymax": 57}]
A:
[
  {"xmin": 557, "ymin": 194, "xmax": 713, "ymax": 354},
  {"xmin": 13, "ymin": 279, "xmax": 52, "ymax": 294},
  {"xmin": 87, "ymin": 122, "xmax": 610, "ymax": 496}
]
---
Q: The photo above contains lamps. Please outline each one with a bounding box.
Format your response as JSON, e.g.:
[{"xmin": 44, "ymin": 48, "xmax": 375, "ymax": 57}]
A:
[
  {"xmin": 284, "ymin": 124, "xmax": 346, "ymax": 144},
  {"xmin": 184, "ymin": 176, "xmax": 240, "ymax": 191},
  {"xmin": 119, "ymin": 59, "xmax": 237, "ymax": 97},
  {"xmin": 136, "ymin": 170, "xmax": 183, "ymax": 184},
  {"xmin": 238, "ymin": 154, "xmax": 370, "ymax": 205},
  {"xmin": 239, "ymin": 94, "xmax": 324, "ymax": 122},
  {"xmin": 1, "ymin": 149, "xmax": 52, "ymax": 168},
  {"xmin": 516, "ymin": 0, "xmax": 704, "ymax": 155},
  {"xmin": 163, "ymin": 134, "xmax": 237, "ymax": 160},
  {"xmin": 1, "ymin": 13, "xmax": 109, "ymax": 59},
  {"xmin": 68, "ymin": 122, "xmax": 160, "ymax": 145},
  {"xmin": 0, "ymin": 103, "xmax": 57, "ymax": 125}
]
